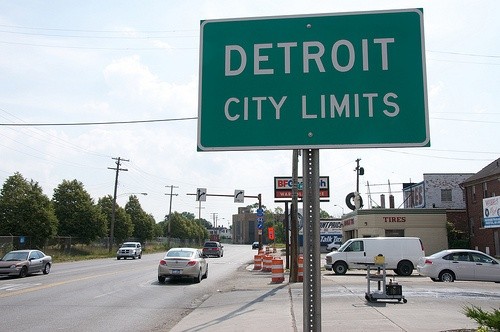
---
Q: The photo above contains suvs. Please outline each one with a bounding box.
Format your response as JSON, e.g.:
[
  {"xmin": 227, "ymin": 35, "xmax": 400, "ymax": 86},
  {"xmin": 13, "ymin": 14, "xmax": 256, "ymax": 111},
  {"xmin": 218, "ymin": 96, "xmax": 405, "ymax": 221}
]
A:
[
  {"xmin": 117, "ymin": 242, "xmax": 142, "ymax": 260},
  {"xmin": 203, "ymin": 241, "xmax": 224, "ymax": 258}
]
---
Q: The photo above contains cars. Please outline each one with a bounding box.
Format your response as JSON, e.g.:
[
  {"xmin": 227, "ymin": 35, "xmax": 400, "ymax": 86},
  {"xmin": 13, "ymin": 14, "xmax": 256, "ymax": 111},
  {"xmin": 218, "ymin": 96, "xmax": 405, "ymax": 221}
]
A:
[
  {"xmin": 326, "ymin": 241, "xmax": 342, "ymax": 252},
  {"xmin": 416, "ymin": 248, "xmax": 500, "ymax": 283},
  {"xmin": 0, "ymin": 249, "xmax": 53, "ymax": 278},
  {"xmin": 252, "ymin": 242, "xmax": 263, "ymax": 249},
  {"xmin": 157, "ymin": 247, "xmax": 208, "ymax": 283}
]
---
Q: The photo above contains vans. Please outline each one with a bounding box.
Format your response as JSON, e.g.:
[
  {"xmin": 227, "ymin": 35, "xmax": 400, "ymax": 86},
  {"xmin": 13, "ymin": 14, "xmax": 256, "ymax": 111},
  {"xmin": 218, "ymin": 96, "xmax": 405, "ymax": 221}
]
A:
[{"xmin": 324, "ymin": 236, "xmax": 425, "ymax": 276}]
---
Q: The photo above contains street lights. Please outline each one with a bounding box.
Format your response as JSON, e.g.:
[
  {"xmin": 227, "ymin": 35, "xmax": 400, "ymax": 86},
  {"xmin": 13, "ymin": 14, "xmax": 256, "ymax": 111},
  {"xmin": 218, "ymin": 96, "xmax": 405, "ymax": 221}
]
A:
[{"xmin": 109, "ymin": 192, "xmax": 148, "ymax": 253}]
